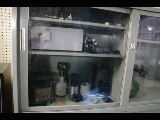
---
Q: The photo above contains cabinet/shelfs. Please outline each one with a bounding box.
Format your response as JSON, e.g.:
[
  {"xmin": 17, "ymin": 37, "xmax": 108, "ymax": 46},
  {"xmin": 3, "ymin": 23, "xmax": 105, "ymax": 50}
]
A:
[{"xmin": 10, "ymin": 7, "xmax": 160, "ymax": 114}]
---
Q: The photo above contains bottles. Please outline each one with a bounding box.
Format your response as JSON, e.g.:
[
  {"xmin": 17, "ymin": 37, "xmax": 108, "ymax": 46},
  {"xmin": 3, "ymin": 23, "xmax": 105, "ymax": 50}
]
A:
[
  {"xmin": 83, "ymin": 37, "xmax": 99, "ymax": 53},
  {"xmin": 139, "ymin": 65, "xmax": 145, "ymax": 77},
  {"xmin": 105, "ymin": 14, "xmax": 112, "ymax": 25},
  {"xmin": 54, "ymin": 70, "xmax": 67, "ymax": 97},
  {"xmin": 44, "ymin": 11, "xmax": 73, "ymax": 21}
]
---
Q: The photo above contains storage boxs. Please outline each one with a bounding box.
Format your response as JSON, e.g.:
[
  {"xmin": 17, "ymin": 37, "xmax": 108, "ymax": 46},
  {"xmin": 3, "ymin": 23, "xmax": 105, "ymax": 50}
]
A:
[{"xmin": 31, "ymin": 24, "xmax": 84, "ymax": 52}]
